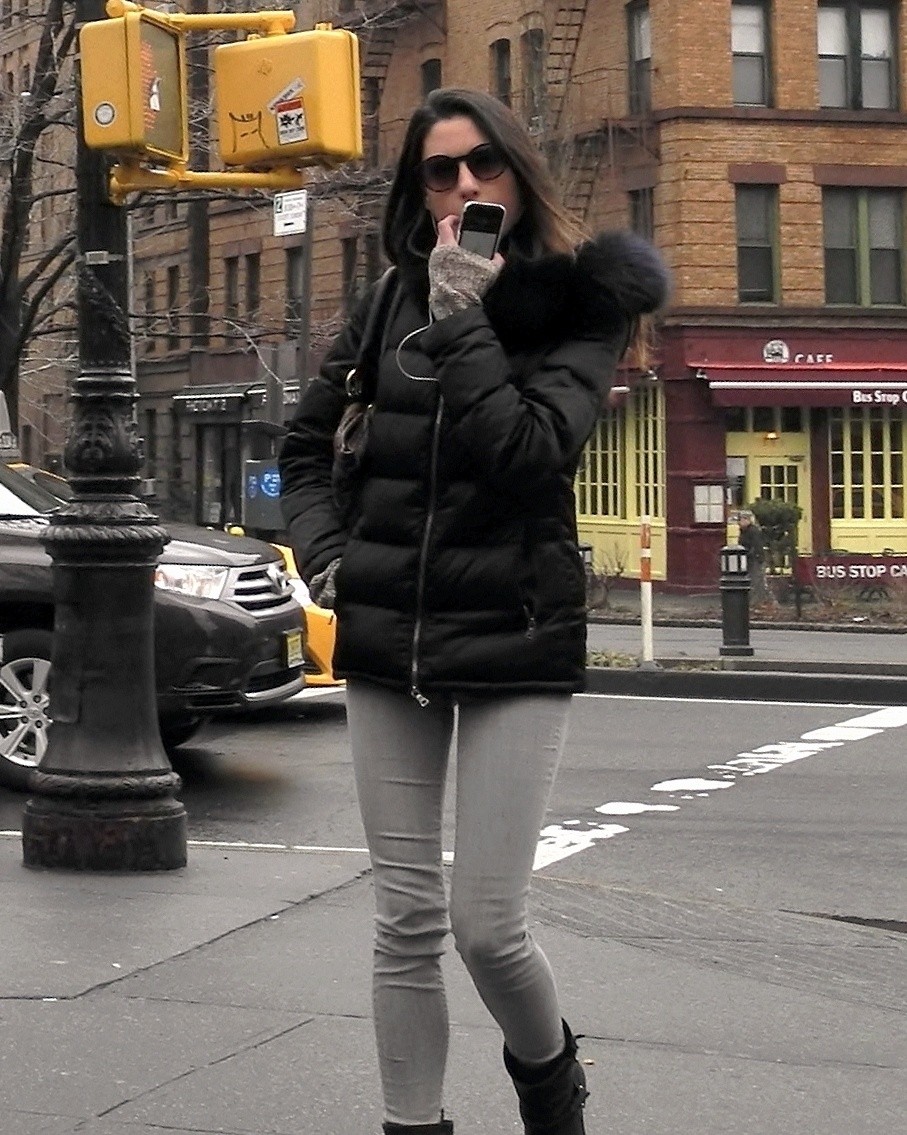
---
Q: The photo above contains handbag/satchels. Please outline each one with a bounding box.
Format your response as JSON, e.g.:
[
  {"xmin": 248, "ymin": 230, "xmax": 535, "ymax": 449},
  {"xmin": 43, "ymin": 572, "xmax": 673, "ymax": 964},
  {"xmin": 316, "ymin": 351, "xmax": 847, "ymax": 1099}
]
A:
[{"xmin": 330, "ymin": 265, "xmax": 410, "ymax": 519}]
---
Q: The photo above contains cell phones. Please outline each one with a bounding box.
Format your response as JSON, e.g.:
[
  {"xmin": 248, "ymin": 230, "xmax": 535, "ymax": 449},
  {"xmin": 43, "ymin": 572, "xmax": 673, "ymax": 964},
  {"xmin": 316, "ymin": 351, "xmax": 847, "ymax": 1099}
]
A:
[{"xmin": 456, "ymin": 201, "xmax": 507, "ymax": 260}]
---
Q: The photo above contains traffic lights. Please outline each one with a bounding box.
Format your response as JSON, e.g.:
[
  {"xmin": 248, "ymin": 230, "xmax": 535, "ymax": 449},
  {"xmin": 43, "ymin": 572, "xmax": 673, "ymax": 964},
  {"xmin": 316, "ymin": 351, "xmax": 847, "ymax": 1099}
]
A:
[{"xmin": 78, "ymin": 9, "xmax": 190, "ymax": 166}]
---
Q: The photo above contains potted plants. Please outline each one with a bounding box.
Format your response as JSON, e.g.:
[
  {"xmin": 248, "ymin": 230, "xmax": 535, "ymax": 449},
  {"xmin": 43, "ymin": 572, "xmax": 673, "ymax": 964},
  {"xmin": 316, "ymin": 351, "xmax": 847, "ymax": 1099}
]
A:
[{"xmin": 752, "ymin": 496, "xmax": 803, "ymax": 599}]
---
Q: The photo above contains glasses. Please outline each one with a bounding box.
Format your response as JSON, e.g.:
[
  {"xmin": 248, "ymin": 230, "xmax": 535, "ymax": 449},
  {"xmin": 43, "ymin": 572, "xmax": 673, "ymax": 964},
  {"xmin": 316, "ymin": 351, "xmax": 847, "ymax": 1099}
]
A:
[{"xmin": 414, "ymin": 139, "xmax": 509, "ymax": 193}]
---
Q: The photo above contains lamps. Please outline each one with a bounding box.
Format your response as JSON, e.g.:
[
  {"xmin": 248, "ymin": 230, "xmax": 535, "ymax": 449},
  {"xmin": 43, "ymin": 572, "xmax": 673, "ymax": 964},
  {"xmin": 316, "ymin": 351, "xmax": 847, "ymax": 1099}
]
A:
[
  {"xmin": 720, "ymin": 545, "xmax": 749, "ymax": 575},
  {"xmin": 780, "ymin": 441, "xmax": 786, "ymax": 458},
  {"xmin": 577, "ymin": 543, "xmax": 592, "ymax": 566}
]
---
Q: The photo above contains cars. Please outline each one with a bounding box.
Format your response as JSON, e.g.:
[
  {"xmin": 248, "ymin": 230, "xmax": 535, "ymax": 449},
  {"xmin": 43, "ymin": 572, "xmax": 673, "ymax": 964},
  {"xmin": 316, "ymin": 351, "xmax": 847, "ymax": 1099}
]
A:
[{"xmin": 6, "ymin": 463, "xmax": 346, "ymax": 689}]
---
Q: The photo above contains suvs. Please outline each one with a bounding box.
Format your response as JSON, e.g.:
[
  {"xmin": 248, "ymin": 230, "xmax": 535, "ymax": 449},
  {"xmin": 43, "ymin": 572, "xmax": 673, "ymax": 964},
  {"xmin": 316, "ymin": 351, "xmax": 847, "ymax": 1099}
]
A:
[{"xmin": 0, "ymin": 461, "xmax": 309, "ymax": 790}]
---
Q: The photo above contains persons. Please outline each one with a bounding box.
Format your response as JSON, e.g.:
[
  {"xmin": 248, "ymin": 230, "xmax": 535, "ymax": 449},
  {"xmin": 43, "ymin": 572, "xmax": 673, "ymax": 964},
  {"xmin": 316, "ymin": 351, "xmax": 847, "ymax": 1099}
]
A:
[
  {"xmin": 733, "ymin": 512, "xmax": 772, "ymax": 607},
  {"xmin": 278, "ymin": 87, "xmax": 670, "ymax": 1135}
]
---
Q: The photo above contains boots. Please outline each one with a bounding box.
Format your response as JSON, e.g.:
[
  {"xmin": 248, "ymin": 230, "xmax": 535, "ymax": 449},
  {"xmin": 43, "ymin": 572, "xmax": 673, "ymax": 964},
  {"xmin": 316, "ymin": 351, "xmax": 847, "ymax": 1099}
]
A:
[
  {"xmin": 502, "ymin": 1015, "xmax": 593, "ymax": 1135},
  {"xmin": 380, "ymin": 1107, "xmax": 455, "ymax": 1135}
]
